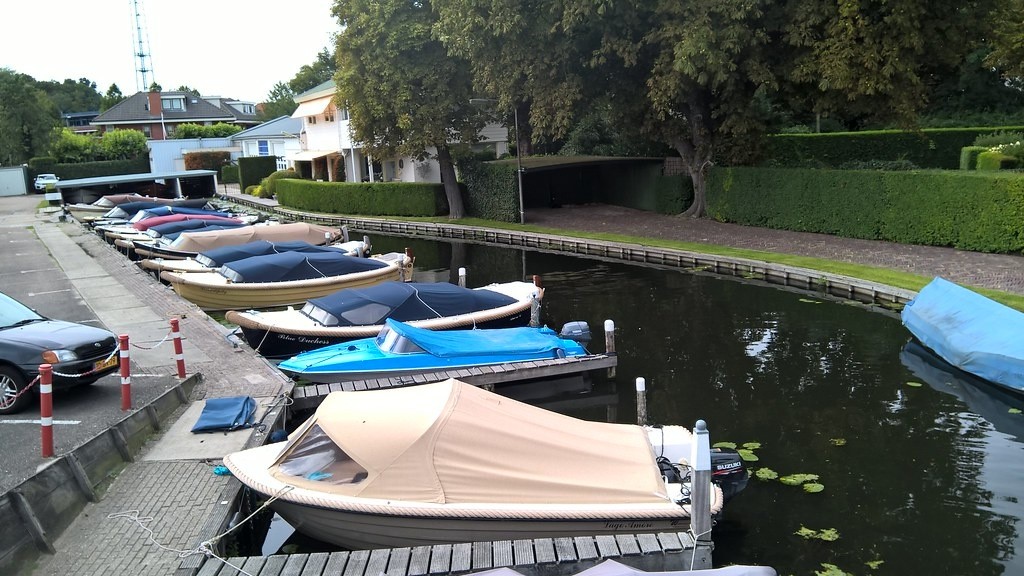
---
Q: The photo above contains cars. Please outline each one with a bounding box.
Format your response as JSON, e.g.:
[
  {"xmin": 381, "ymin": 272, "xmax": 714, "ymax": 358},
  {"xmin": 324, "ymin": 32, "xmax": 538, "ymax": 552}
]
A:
[
  {"xmin": 0, "ymin": 292, "xmax": 124, "ymax": 412},
  {"xmin": 34, "ymin": 174, "xmax": 60, "ymax": 193}
]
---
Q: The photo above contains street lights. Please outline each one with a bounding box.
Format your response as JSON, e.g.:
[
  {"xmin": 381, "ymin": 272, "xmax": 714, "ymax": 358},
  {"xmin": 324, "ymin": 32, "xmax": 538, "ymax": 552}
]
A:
[{"xmin": 469, "ymin": 98, "xmax": 525, "ymax": 224}]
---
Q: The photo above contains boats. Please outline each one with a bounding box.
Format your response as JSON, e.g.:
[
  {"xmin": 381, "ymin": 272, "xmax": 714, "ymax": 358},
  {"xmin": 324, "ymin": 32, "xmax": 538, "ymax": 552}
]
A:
[
  {"xmin": 275, "ymin": 317, "xmax": 597, "ymax": 382},
  {"xmin": 159, "ymin": 248, "xmax": 418, "ymax": 313},
  {"xmin": 66, "ymin": 192, "xmax": 374, "ymax": 271},
  {"xmin": 219, "ymin": 376, "xmax": 752, "ymax": 549},
  {"xmin": 222, "ymin": 281, "xmax": 546, "ymax": 348}
]
